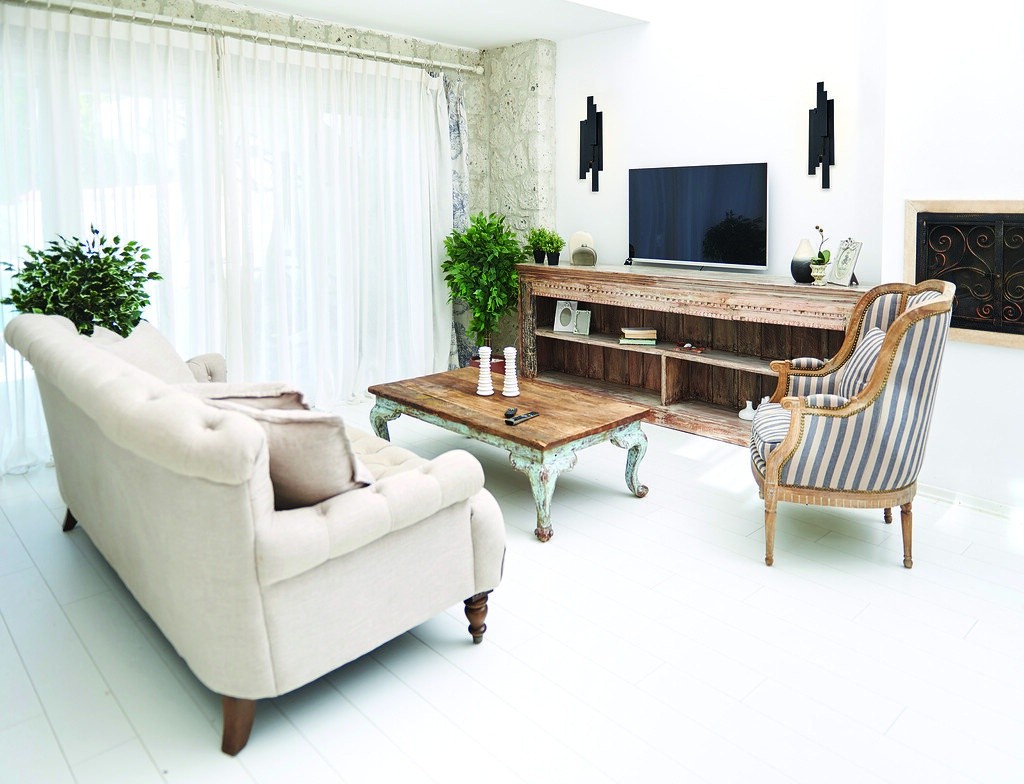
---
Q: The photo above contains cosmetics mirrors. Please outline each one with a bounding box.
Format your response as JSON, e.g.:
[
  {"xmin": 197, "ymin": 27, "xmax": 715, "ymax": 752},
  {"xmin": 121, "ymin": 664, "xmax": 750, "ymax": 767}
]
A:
[{"xmin": 553, "ymin": 301, "xmax": 591, "ymax": 335}]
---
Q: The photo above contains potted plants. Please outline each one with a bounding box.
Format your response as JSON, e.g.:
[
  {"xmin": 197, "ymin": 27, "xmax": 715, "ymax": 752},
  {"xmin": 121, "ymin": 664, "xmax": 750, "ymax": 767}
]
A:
[
  {"xmin": 544, "ymin": 231, "xmax": 566, "ymax": 265},
  {"xmin": 439, "ymin": 211, "xmax": 527, "ymax": 377},
  {"xmin": 525, "ymin": 226, "xmax": 546, "ymax": 263},
  {"xmin": 809, "ymin": 226, "xmax": 832, "ymax": 286}
]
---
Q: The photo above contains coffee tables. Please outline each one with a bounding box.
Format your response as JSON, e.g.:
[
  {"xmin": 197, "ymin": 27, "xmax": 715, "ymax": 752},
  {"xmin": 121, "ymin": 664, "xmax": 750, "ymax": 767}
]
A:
[{"xmin": 367, "ymin": 366, "xmax": 651, "ymax": 544}]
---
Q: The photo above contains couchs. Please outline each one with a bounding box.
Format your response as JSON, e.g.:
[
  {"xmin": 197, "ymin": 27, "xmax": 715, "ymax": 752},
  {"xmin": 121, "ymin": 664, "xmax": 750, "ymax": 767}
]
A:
[
  {"xmin": 749, "ymin": 280, "xmax": 957, "ymax": 570},
  {"xmin": 5, "ymin": 314, "xmax": 507, "ymax": 757}
]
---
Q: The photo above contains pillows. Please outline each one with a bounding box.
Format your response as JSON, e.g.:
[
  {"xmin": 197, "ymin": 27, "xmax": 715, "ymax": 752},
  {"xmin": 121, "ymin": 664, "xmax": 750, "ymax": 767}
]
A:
[
  {"xmin": 162, "ymin": 381, "xmax": 375, "ymax": 512},
  {"xmin": 837, "ymin": 327, "xmax": 887, "ymax": 400}
]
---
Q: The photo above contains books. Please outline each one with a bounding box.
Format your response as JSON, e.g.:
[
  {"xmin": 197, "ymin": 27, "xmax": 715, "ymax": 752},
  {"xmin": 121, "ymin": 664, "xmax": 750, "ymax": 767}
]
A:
[{"xmin": 619, "ymin": 328, "xmax": 657, "ymax": 345}]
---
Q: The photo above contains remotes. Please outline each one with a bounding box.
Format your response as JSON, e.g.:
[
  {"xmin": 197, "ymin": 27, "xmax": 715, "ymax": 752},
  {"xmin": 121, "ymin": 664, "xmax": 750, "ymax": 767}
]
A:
[
  {"xmin": 505, "ymin": 412, "xmax": 540, "ymax": 425},
  {"xmin": 504, "ymin": 408, "xmax": 518, "ymax": 418}
]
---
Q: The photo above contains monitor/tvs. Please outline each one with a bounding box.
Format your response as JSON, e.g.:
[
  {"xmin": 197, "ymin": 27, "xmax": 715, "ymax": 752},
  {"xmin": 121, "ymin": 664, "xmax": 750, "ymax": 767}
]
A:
[{"xmin": 629, "ymin": 163, "xmax": 769, "ymax": 270}]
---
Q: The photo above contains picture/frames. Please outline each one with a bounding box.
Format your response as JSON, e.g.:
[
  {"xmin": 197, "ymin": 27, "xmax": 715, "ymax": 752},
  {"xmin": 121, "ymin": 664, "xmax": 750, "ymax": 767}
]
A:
[
  {"xmin": 553, "ymin": 301, "xmax": 578, "ymax": 333},
  {"xmin": 573, "ymin": 310, "xmax": 591, "ymax": 335},
  {"xmin": 827, "ymin": 237, "xmax": 862, "ymax": 286}
]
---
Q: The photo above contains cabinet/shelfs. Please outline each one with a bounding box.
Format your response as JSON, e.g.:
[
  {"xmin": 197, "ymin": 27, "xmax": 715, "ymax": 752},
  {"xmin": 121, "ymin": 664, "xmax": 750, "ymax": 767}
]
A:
[{"xmin": 512, "ymin": 262, "xmax": 867, "ymax": 448}]
